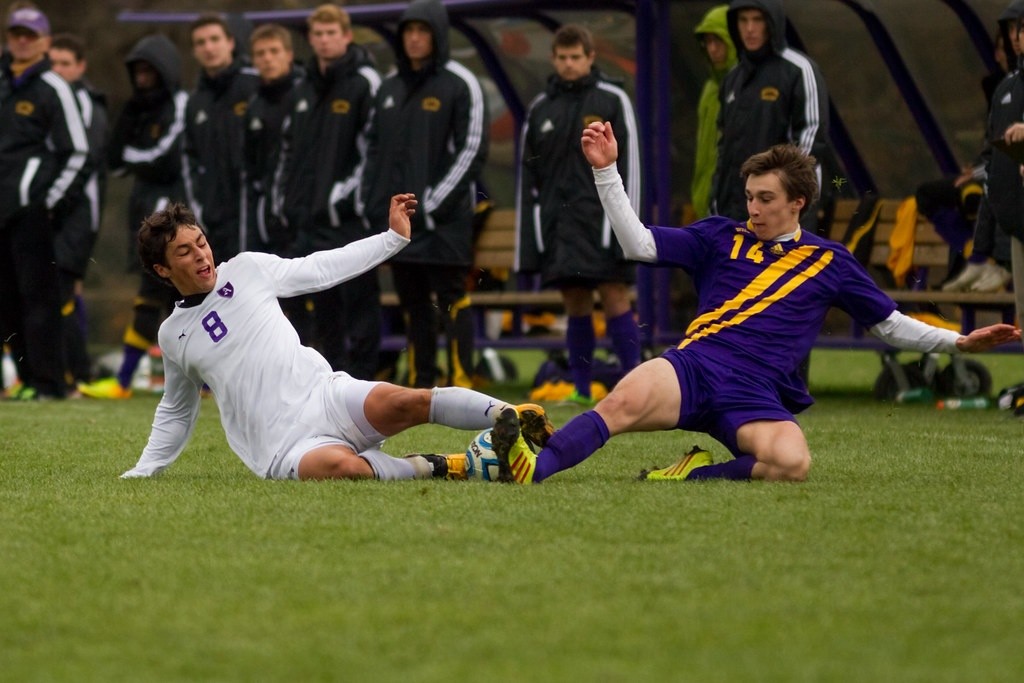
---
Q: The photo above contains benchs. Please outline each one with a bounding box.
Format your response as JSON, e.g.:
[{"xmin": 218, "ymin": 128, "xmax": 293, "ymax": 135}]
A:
[{"xmin": 374, "ymin": 193, "xmax": 1024, "ymax": 386}]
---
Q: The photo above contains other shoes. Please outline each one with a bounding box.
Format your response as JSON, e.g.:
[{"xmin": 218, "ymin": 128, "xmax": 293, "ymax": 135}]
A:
[
  {"xmin": 943, "ymin": 262, "xmax": 984, "ymax": 291},
  {"xmin": 971, "ymin": 266, "xmax": 1011, "ymax": 289}
]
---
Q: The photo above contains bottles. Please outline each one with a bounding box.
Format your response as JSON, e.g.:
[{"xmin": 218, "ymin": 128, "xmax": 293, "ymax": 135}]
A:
[
  {"xmin": 895, "ymin": 389, "xmax": 926, "ymax": 403},
  {"xmin": 935, "ymin": 397, "xmax": 990, "ymax": 412}
]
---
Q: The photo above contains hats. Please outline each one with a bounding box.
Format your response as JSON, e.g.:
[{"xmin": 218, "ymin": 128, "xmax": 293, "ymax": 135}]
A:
[{"xmin": 5, "ymin": 8, "xmax": 51, "ymax": 37}]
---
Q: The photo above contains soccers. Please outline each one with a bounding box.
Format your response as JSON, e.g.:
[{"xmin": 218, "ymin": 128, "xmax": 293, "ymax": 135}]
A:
[{"xmin": 464, "ymin": 427, "xmax": 537, "ymax": 482}]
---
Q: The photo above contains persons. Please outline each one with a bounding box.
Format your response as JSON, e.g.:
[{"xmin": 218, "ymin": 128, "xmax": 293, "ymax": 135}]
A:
[
  {"xmin": 490, "ymin": 120, "xmax": 1024, "ymax": 485},
  {"xmin": 513, "ymin": 24, "xmax": 643, "ymax": 403},
  {"xmin": 1, "ymin": 7, "xmax": 110, "ymax": 400},
  {"xmin": 77, "ymin": 31, "xmax": 191, "ymax": 401},
  {"xmin": 915, "ymin": 1, "xmax": 1023, "ymax": 295},
  {"xmin": 682, "ymin": 0, "xmax": 831, "ymax": 225},
  {"xmin": 119, "ymin": 192, "xmax": 556, "ymax": 481},
  {"xmin": 354, "ymin": 1, "xmax": 488, "ymax": 392},
  {"xmin": 181, "ymin": 2, "xmax": 382, "ymax": 378}
]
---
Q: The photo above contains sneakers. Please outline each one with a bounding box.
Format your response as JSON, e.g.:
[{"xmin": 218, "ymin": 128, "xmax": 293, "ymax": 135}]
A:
[
  {"xmin": 423, "ymin": 452, "xmax": 469, "ymax": 479},
  {"xmin": 518, "ymin": 402, "xmax": 556, "ymax": 445},
  {"xmin": 557, "ymin": 392, "xmax": 594, "ymax": 407},
  {"xmin": 75, "ymin": 378, "xmax": 134, "ymax": 399},
  {"xmin": 636, "ymin": 445, "xmax": 713, "ymax": 480},
  {"xmin": 492, "ymin": 405, "xmax": 538, "ymax": 486}
]
